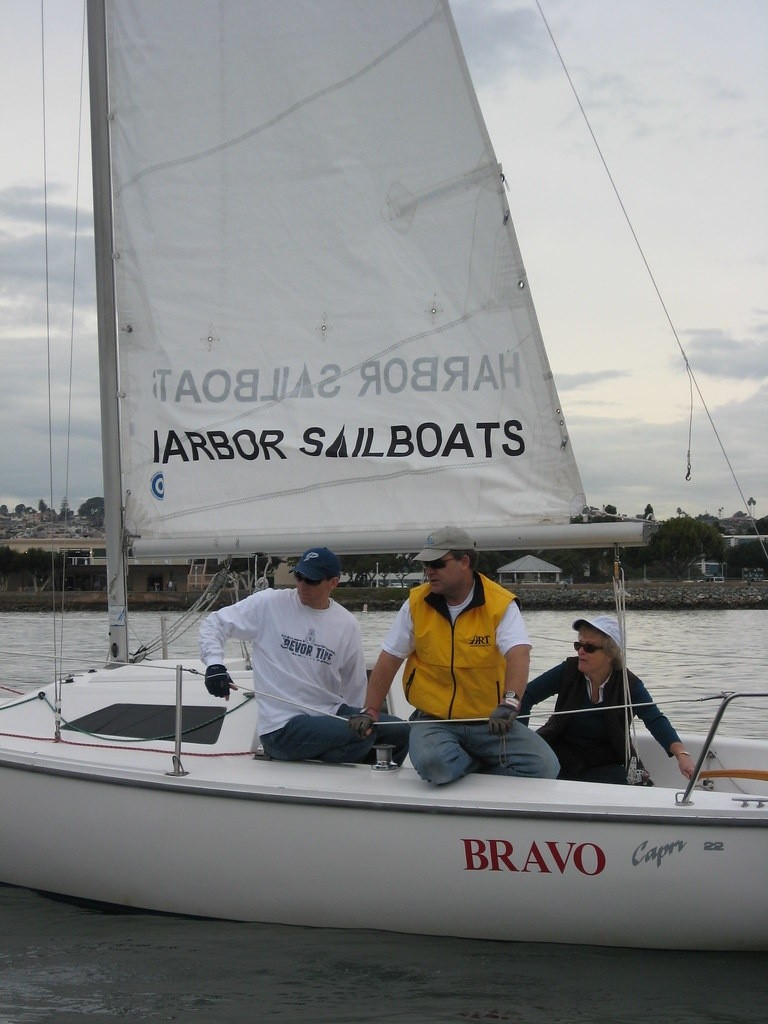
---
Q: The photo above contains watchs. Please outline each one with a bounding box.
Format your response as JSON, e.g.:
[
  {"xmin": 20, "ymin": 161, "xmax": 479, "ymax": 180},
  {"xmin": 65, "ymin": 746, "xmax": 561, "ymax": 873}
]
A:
[
  {"xmin": 359, "ymin": 707, "xmax": 378, "ymax": 717},
  {"xmin": 503, "ymin": 690, "xmax": 520, "ymax": 700}
]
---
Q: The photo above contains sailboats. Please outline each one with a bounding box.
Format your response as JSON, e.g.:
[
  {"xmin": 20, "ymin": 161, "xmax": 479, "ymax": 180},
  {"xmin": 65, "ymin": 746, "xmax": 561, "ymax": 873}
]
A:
[{"xmin": 0, "ymin": 0, "xmax": 768, "ymax": 954}]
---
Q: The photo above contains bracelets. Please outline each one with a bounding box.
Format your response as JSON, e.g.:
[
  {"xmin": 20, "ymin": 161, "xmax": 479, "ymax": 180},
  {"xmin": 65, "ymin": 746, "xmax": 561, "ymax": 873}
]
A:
[{"xmin": 676, "ymin": 751, "xmax": 690, "ymax": 757}]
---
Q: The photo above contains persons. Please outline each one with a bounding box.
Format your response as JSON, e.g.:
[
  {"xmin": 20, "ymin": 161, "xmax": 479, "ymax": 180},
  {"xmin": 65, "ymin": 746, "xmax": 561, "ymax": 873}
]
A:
[
  {"xmin": 349, "ymin": 524, "xmax": 562, "ymax": 786},
  {"xmin": 167, "ymin": 577, "xmax": 173, "ymax": 591},
  {"xmin": 519, "ymin": 617, "xmax": 696, "ymax": 787},
  {"xmin": 198, "ymin": 546, "xmax": 412, "ymax": 769}
]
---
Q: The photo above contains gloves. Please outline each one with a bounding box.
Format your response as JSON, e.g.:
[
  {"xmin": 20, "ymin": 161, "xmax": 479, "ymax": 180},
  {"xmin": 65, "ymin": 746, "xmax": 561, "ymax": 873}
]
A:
[{"xmin": 490, "ymin": 690, "xmax": 521, "ymax": 733}]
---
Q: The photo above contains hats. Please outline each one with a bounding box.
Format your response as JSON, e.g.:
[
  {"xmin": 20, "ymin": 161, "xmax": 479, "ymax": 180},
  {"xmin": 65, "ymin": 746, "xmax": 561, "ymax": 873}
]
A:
[
  {"xmin": 412, "ymin": 526, "xmax": 476, "ymax": 561},
  {"xmin": 289, "ymin": 547, "xmax": 341, "ymax": 581},
  {"xmin": 571, "ymin": 616, "xmax": 621, "ymax": 649}
]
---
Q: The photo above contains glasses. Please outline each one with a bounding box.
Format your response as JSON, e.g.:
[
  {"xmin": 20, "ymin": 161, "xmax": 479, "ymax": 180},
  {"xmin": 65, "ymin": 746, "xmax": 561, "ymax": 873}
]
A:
[
  {"xmin": 421, "ymin": 555, "xmax": 463, "ymax": 570},
  {"xmin": 294, "ymin": 571, "xmax": 337, "ymax": 586},
  {"xmin": 574, "ymin": 642, "xmax": 609, "ymax": 653}
]
